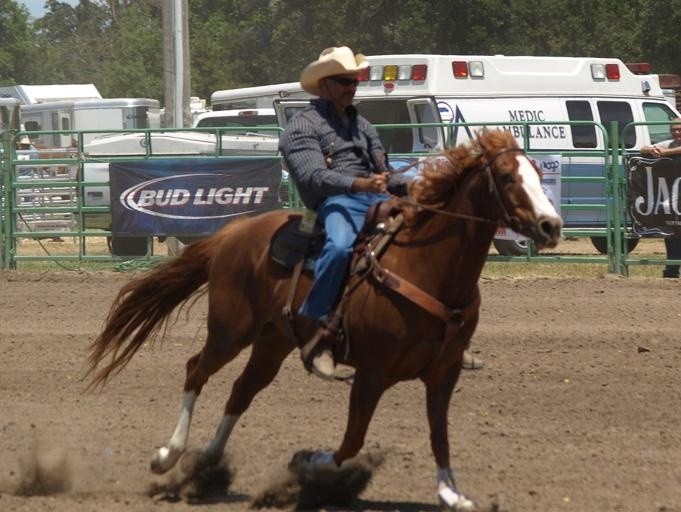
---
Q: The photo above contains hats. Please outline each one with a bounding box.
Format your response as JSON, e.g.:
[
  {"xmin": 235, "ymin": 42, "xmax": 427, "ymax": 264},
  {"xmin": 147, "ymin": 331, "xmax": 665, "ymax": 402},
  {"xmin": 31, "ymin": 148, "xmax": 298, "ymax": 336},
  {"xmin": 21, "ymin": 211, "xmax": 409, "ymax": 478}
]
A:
[{"xmin": 300, "ymin": 45, "xmax": 370, "ymax": 97}]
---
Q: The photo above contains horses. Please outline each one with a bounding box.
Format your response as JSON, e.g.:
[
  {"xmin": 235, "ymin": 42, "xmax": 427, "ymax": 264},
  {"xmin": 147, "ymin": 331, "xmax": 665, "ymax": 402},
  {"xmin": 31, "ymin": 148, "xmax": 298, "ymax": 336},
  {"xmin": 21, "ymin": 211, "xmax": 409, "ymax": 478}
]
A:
[{"xmin": 79, "ymin": 126, "xmax": 563, "ymax": 512}]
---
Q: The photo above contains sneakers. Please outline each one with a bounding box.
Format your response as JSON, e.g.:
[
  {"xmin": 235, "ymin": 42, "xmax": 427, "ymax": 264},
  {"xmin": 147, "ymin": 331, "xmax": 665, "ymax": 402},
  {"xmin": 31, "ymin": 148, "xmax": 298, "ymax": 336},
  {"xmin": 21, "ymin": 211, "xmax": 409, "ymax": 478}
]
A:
[
  {"xmin": 462, "ymin": 351, "xmax": 485, "ymax": 370},
  {"xmin": 298, "ymin": 309, "xmax": 338, "ymax": 379}
]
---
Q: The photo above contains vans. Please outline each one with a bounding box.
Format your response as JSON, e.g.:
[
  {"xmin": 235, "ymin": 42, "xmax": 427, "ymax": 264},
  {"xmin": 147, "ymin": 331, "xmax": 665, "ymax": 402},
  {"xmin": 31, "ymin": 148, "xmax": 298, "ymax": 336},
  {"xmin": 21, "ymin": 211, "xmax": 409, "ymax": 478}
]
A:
[{"xmin": 0, "ymin": 51, "xmax": 681, "ymax": 258}]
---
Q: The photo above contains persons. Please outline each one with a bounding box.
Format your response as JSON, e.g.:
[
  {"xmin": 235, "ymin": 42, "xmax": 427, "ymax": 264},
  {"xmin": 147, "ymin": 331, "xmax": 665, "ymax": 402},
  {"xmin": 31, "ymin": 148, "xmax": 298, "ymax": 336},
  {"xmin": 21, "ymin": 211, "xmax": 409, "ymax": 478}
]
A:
[
  {"xmin": 15, "ymin": 136, "xmax": 43, "ymax": 202},
  {"xmin": 277, "ymin": 40, "xmax": 487, "ymax": 382},
  {"xmin": 638, "ymin": 117, "xmax": 681, "ymax": 279}
]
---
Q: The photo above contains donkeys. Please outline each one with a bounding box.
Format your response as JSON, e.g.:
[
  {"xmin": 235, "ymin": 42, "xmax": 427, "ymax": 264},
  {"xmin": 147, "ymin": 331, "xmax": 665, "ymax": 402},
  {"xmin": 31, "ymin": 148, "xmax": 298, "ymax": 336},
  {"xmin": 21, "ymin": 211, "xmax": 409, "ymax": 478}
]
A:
[{"xmin": 32, "ymin": 144, "xmax": 78, "ymax": 220}]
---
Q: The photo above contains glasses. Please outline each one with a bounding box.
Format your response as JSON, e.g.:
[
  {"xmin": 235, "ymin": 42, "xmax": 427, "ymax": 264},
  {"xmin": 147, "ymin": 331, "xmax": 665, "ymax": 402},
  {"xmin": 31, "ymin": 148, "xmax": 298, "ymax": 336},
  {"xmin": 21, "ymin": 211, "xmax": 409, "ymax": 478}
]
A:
[{"xmin": 329, "ymin": 76, "xmax": 361, "ymax": 86}]
